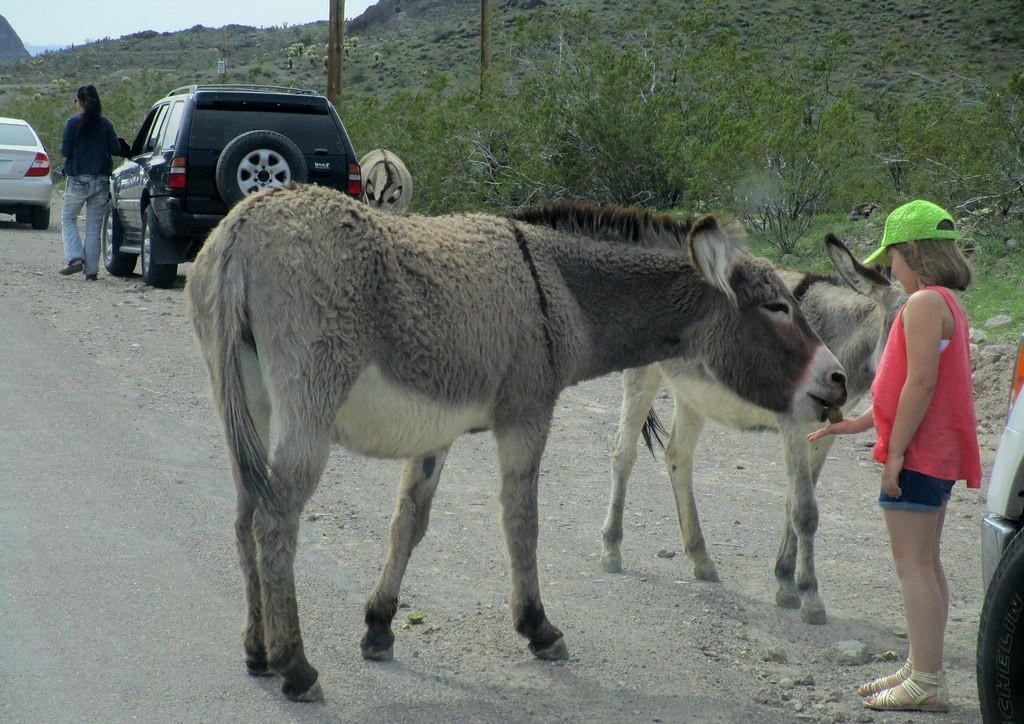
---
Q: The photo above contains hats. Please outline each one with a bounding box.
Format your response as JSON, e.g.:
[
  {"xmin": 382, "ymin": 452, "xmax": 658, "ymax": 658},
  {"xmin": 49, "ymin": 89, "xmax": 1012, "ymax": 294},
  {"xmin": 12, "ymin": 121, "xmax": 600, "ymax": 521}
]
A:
[{"xmin": 862, "ymin": 199, "xmax": 960, "ymax": 266}]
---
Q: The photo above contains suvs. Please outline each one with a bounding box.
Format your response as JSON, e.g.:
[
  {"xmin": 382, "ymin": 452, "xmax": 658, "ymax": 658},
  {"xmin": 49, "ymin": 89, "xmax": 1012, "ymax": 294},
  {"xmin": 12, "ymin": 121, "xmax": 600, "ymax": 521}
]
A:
[{"xmin": 100, "ymin": 85, "xmax": 360, "ymax": 289}]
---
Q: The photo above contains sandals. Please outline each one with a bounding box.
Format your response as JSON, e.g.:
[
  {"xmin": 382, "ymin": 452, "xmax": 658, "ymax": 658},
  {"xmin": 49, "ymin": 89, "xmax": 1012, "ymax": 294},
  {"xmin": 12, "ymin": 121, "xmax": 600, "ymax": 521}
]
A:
[{"xmin": 857, "ymin": 658, "xmax": 950, "ymax": 712}]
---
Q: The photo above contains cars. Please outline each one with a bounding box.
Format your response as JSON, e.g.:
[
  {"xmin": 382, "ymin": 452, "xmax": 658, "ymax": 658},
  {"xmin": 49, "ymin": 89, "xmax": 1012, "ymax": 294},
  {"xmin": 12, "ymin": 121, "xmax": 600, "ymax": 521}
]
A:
[
  {"xmin": 0, "ymin": 117, "xmax": 50, "ymax": 231},
  {"xmin": 977, "ymin": 332, "xmax": 1024, "ymax": 724}
]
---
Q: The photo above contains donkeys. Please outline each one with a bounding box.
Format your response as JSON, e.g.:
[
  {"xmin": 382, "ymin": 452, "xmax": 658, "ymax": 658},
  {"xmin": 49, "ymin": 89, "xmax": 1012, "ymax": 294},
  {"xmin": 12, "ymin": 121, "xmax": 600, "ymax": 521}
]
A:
[
  {"xmin": 599, "ymin": 232, "xmax": 980, "ymax": 627},
  {"xmin": 184, "ymin": 178, "xmax": 850, "ymax": 704}
]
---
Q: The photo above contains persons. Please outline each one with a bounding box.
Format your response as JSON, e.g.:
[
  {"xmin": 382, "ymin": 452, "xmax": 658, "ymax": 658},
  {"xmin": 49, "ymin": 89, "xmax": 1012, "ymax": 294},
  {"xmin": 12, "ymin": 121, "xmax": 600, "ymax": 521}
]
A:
[
  {"xmin": 60, "ymin": 83, "xmax": 121, "ymax": 282},
  {"xmin": 806, "ymin": 201, "xmax": 981, "ymax": 712}
]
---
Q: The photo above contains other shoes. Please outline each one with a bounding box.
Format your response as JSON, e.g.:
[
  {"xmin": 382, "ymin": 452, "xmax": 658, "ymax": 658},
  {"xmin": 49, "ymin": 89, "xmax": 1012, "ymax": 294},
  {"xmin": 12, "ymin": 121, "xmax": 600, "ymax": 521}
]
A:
[
  {"xmin": 86, "ymin": 274, "xmax": 98, "ymax": 281},
  {"xmin": 59, "ymin": 264, "xmax": 83, "ymax": 275}
]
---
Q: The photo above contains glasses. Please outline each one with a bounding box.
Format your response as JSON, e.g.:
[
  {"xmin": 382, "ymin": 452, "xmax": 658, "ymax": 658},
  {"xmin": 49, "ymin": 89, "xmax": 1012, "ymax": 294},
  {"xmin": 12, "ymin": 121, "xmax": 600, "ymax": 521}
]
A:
[{"xmin": 74, "ymin": 97, "xmax": 80, "ymax": 104}]
